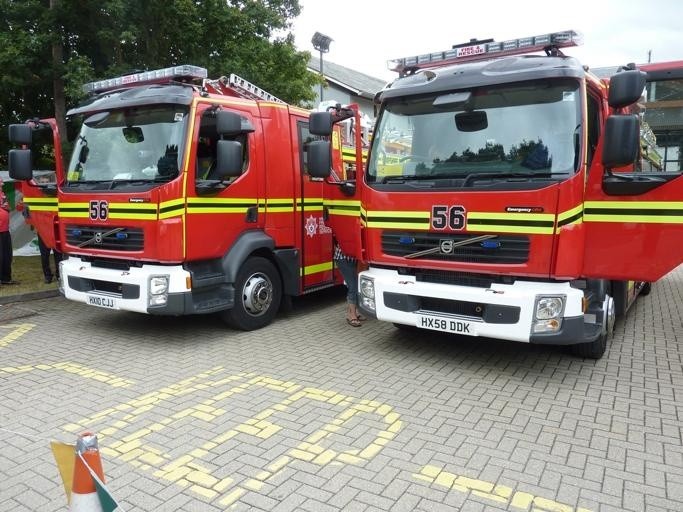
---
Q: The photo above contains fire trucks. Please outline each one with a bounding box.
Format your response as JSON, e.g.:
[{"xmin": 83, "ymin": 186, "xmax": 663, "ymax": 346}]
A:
[
  {"xmin": 7, "ymin": 64, "xmax": 413, "ymax": 333},
  {"xmin": 307, "ymin": 30, "xmax": 683, "ymax": 358}
]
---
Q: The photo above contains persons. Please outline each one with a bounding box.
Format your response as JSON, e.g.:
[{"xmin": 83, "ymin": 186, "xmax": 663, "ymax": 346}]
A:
[
  {"xmin": 333, "ymin": 243, "xmax": 367, "ymax": 328},
  {"xmin": 0, "ymin": 176, "xmax": 20, "ymax": 286},
  {"xmin": 27, "ymin": 174, "xmax": 63, "ymax": 284}
]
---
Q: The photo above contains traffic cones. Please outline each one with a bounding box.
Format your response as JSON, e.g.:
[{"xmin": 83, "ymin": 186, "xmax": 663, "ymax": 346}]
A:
[{"xmin": 66, "ymin": 432, "xmax": 105, "ymax": 512}]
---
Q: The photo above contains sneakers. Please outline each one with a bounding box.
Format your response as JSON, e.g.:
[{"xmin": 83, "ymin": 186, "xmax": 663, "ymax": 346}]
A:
[
  {"xmin": 1, "ymin": 279, "xmax": 19, "ymax": 285},
  {"xmin": 44, "ymin": 276, "xmax": 55, "ymax": 284}
]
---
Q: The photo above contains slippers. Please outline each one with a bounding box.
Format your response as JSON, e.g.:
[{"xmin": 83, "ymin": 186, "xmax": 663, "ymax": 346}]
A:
[{"xmin": 347, "ymin": 313, "xmax": 365, "ymax": 327}]
